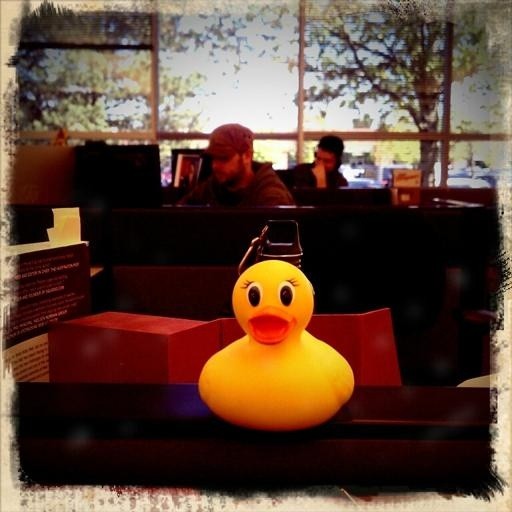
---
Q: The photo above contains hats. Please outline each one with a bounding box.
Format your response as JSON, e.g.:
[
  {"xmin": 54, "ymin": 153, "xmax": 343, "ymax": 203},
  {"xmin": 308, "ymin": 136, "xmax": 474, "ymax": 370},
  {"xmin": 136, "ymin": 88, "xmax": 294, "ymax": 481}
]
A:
[{"xmin": 204, "ymin": 124, "xmax": 253, "ymax": 158}]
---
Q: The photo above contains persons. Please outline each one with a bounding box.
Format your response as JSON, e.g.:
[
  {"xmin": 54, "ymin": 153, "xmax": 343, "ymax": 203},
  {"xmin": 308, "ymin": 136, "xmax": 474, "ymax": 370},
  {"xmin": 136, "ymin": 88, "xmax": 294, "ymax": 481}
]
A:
[
  {"xmin": 276, "ymin": 137, "xmax": 348, "ymax": 189},
  {"xmin": 176, "ymin": 123, "xmax": 298, "ymax": 207}
]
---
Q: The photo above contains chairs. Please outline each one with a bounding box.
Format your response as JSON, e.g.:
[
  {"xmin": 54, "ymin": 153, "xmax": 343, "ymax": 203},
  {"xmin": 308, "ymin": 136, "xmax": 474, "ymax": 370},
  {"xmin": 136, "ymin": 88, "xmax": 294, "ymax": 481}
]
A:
[{"xmin": 109, "ymin": 263, "xmax": 248, "ymax": 321}]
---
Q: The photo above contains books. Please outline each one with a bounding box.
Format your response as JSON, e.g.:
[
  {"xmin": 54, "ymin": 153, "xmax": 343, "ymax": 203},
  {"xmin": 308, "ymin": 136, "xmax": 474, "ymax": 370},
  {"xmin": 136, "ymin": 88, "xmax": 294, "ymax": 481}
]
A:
[
  {"xmin": 391, "ymin": 168, "xmax": 423, "ymax": 205},
  {"xmin": 2, "ymin": 243, "xmax": 92, "ymax": 382}
]
